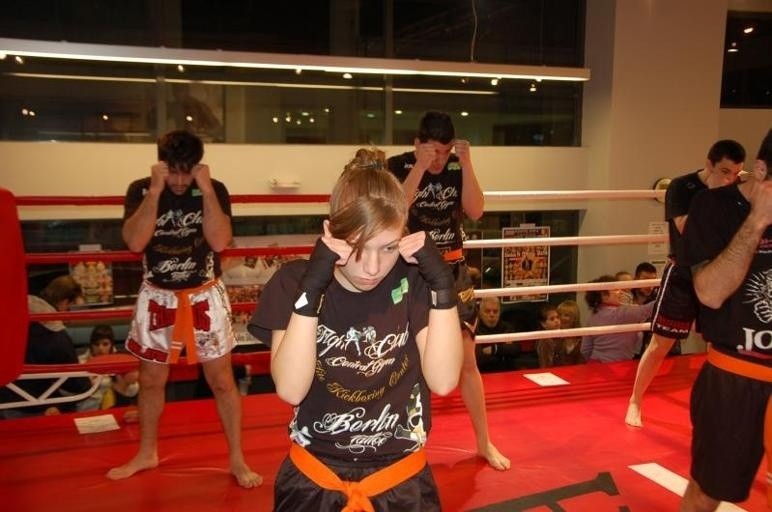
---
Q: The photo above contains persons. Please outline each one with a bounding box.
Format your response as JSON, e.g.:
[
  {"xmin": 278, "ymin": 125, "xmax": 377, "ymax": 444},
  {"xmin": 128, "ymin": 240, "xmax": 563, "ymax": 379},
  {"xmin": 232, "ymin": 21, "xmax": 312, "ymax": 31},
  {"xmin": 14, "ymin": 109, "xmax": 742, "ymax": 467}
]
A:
[
  {"xmin": 622, "ymin": 139, "xmax": 748, "ymax": 427},
  {"xmin": 102, "ymin": 129, "xmax": 265, "ymax": 491},
  {"xmin": 386, "ymin": 108, "xmax": 514, "ymax": 470},
  {"xmin": 248, "ymin": 146, "xmax": 466, "ymax": 512},
  {"xmin": 475, "ymin": 299, "xmax": 513, "ymax": 372},
  {"xmin": 164, "ymin": 285, "xmax": 276, "ymax": 402},
  {"xmin": 0, "ymin": 276, "xmax": 140, "ymax": 417},
  {"xmin": 537, "ymin": 262, "xmax": 657, "ymax": 368},
  {"xmin": 680, "ymin": 129, "xmax": 772, "ymax": 512}
]
